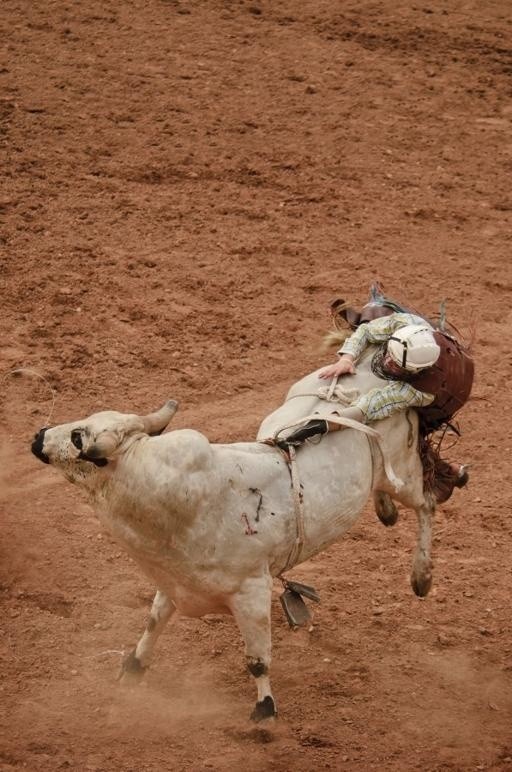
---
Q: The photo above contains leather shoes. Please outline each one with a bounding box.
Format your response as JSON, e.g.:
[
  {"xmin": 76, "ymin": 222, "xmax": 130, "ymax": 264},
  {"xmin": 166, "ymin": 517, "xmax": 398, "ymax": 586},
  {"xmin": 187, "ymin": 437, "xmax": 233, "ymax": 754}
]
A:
[{"xmin": 436, "ymin": 466, "xmax": 469, "ymax": 505}]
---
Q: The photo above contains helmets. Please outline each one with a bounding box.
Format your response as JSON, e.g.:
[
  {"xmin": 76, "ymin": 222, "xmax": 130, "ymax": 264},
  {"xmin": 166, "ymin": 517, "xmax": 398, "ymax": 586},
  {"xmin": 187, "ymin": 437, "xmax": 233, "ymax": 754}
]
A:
[{"xmin": 371, "ymin": 325, "xmax": 440, "ymax": 380}]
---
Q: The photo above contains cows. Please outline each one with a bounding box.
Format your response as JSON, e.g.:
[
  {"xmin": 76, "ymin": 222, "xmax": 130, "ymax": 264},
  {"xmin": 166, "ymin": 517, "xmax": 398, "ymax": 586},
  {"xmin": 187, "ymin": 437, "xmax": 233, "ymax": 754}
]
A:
[{"xmin": 31, "ymin": 315, "xmax": 434, "ymax": 728}]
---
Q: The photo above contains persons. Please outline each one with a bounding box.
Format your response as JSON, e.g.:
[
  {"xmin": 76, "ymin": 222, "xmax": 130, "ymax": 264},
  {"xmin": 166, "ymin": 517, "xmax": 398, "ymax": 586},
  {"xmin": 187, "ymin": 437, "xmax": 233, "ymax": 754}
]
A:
[{"xmin": 318, "ymin": 304, "xmax": 476, "ymax": 503}]
